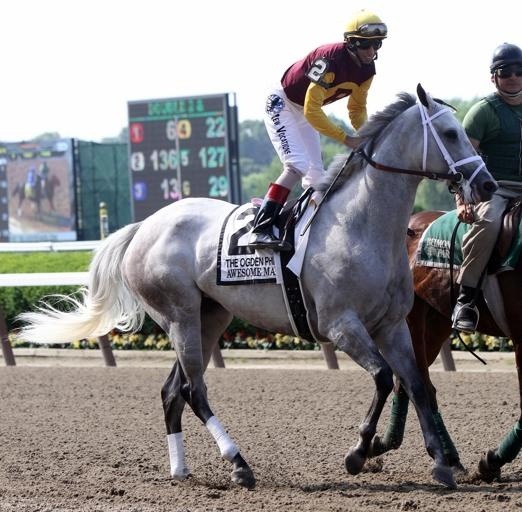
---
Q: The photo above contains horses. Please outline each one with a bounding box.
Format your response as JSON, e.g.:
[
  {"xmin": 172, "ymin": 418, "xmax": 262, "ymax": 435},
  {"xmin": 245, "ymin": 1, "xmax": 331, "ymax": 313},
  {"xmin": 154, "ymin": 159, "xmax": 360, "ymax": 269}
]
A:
[
  {"xmin": 4, "ymin": 84, "xmax": 497, "ymax": 488},
  {"xmin": 367, "ymin": 210, "xmax": 522, "ymax": 483},
  {"xmin": 12, "ymin": 175, "xmax": 42, "ymax": 217},
  {"xmin": 41, "ymin": 175, "xmax": 61, "ymax": 210}
]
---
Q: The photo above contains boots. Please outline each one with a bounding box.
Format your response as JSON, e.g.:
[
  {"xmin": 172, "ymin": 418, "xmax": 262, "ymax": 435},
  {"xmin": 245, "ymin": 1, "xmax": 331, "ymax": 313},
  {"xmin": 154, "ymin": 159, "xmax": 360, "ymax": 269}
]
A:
[
  {"xmin": 248, "ymin": 182, "xmax": 292, "ymax": 250},
  {"xmin": 451, "ymin": 285, "xmax": 478, "ymax": 332}
]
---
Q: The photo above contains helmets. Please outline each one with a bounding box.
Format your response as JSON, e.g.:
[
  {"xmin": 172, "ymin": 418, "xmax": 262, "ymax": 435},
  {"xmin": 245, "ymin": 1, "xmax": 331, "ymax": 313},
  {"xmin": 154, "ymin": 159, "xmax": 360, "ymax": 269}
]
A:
[
  {"xmin": 490, "ymin": 43, "xmax": 522, "ymax": 69},
  {"xmin": 345, "ymin": 13, "xmax": 387, "ymax": 37}
]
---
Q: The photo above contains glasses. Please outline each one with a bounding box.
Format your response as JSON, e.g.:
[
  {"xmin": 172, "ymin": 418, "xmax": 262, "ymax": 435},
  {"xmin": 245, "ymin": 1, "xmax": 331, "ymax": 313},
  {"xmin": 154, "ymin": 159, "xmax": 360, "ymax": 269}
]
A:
[
  {"xmin": 344, "ymin": 23, "xmax": 388, "ymax": 37},
  {"xmin": 354, "ymin": 40, "xmax": 383, "ymax": 49},
  {"xmin": 491, "ymin": 65, "xmax": 522, "ymax": 78}
]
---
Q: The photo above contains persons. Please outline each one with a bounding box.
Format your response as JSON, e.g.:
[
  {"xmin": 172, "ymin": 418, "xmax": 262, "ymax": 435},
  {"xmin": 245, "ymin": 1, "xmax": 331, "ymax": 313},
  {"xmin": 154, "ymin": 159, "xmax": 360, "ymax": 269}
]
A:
[
  {"xmin": 39, "ymin": 161, "xmax": 50, "ymax": 189},
  {"xmin": 24, "ymin": 164, "xmax": 40, "ymax": 203},
  {"xmin": 448, "ymin": 42, "xmax": 522, "ymax": 336},
  {"xmin": 247, "ymin": 12, "xmax": 388, "ymax": 249}
]
